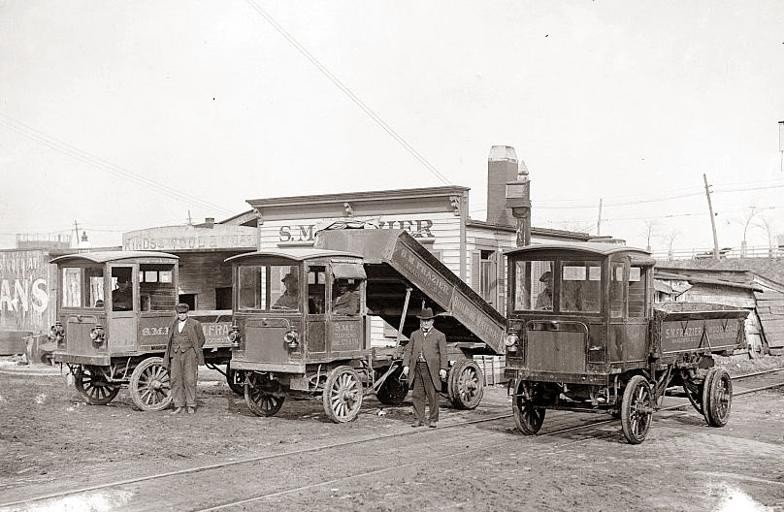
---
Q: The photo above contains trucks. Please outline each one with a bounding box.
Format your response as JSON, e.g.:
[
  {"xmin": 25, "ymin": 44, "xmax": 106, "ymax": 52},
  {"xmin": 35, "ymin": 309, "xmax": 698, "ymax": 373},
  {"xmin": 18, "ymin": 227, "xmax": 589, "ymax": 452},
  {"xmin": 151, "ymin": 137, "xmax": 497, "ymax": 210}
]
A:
[
  {"xmin": 495, "ymin": 236, "xmax": 756, "ymax": 440},
  {"xmin": 44, "ymin": 245, "xmax": 247, "ymax": 412},
  {"xmin": 227, "ymin": 220, "xmax": 512, "ymax": 421}
]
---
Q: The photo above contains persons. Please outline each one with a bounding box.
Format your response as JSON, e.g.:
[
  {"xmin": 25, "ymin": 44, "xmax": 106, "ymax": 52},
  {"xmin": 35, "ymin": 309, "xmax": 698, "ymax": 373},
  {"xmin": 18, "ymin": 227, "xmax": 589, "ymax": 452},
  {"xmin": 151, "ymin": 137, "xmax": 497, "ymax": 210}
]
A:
[
  {"xmin": 273, "ymin": 274, "xmax": 315, "ymax": 315},
  {"xmin": 114, "ymin": 276, "xmax": 142, "ymax": 310},
  {"xmin": 401, "ymin": 307, "xmax": 448, "ymax": 428},
  {"xmin": 162, "ymin": 303, "xmax": 205, "ymax": 414},
  {"xmin": 331, "ymin": 280, "xmax": 357, "ymax": 316},
  {"xmin": 535, "ymin": 271, "xmax": 583, "ymax": 311}
]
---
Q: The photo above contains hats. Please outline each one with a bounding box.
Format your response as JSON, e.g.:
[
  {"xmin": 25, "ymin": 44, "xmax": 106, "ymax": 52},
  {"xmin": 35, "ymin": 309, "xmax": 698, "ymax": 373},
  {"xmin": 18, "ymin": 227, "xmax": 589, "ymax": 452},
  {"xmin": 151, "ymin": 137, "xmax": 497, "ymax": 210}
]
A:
[
  {"xmin": 336, "ymin": 278, "xmax": 351, "ymax": 287},
  {"xmin": 281, "ymin": 273, "xmax": 298, "ymax": 284},
  {"xmin": 415, "ymin": 307, "xmax": 441, "ymax": 321},
  {"xmin": 117, "ymin": 276, "xmax": 128, "ymax": 282},
  {"xmin": 173, "ymin": 302, "xmax": 189, "ymax": 313},
  {"xmin": 537, "ymin": 271, "xmax": 554, "ymax": 282}
]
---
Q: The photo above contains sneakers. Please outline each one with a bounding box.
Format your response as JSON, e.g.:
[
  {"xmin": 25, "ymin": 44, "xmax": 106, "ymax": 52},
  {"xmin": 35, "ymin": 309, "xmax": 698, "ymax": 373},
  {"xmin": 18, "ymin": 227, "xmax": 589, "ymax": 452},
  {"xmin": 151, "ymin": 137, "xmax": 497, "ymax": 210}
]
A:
[
  {"xmin": 429, "ymin": 419, "xmax": 438, "ymax": 427},
  {"xmin": 411, "ymin": 420, "xmax": 426, "ymax": 428}
]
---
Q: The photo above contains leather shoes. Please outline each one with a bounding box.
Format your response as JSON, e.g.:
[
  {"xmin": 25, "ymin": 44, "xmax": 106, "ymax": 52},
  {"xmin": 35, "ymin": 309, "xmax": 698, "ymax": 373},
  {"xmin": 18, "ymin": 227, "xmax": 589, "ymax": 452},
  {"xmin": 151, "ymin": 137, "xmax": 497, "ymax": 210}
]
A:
[{"xmin": 170, "ymin": 407, "xmax": 195, "ymax": 416}]
